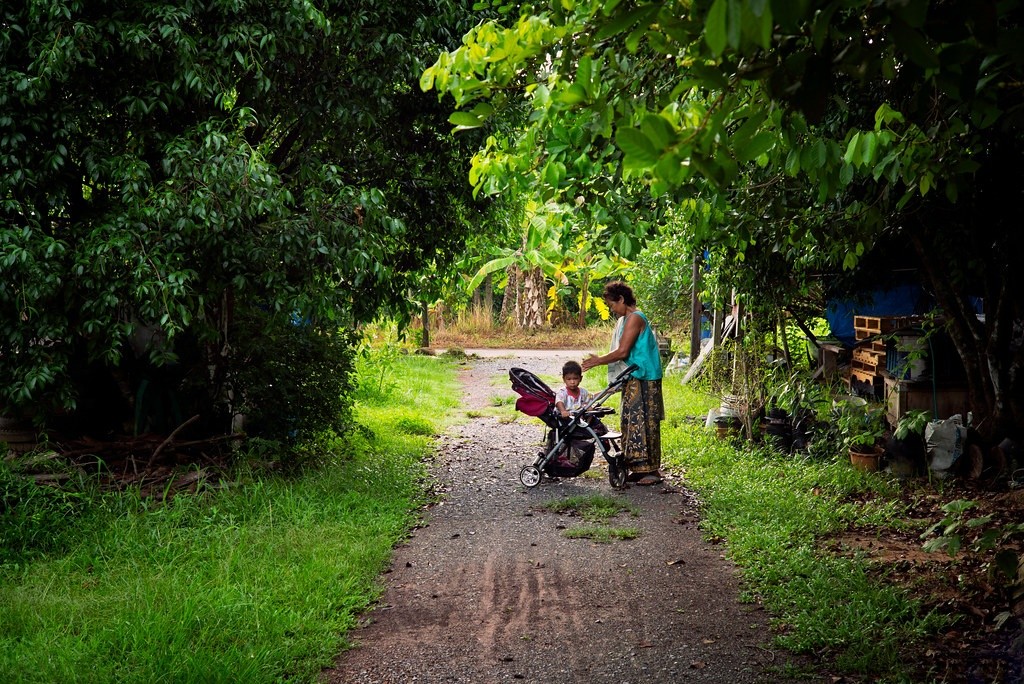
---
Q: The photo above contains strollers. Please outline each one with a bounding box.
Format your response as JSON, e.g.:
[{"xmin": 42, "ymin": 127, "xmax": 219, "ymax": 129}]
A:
[{"xmin": 508, "ymin": 363, "xmax": 641, "ymax": 489}]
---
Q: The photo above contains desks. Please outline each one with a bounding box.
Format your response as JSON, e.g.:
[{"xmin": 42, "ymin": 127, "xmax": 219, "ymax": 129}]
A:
[{"xmin": 879, "ymin": 369, "xmax": 974, "ymax": 435}]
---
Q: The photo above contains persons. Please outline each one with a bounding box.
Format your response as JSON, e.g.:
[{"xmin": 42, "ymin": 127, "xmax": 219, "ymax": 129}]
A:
[
  {"xmin": 554, "ymin": 360, "xmax": 616, "ymax": 427},
  {"xmin": 581, "ymin": 281, "xmax": 666, "ymax": 486}
]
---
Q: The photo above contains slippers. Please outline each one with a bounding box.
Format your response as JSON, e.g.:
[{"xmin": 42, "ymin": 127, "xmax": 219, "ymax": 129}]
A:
[
  {"xmin": 627, "ymin": 471, "xmax": 646, "ymax": 481},
  {"xmin": 637, "ymin": 475, "xmax": 664, "ymax": 486}
]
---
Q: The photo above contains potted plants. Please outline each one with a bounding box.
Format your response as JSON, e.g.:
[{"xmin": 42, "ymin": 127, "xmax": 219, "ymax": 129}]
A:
[{"xmin": 829, "ymin": 400, "xmax": 888, "ymax": 471}]
